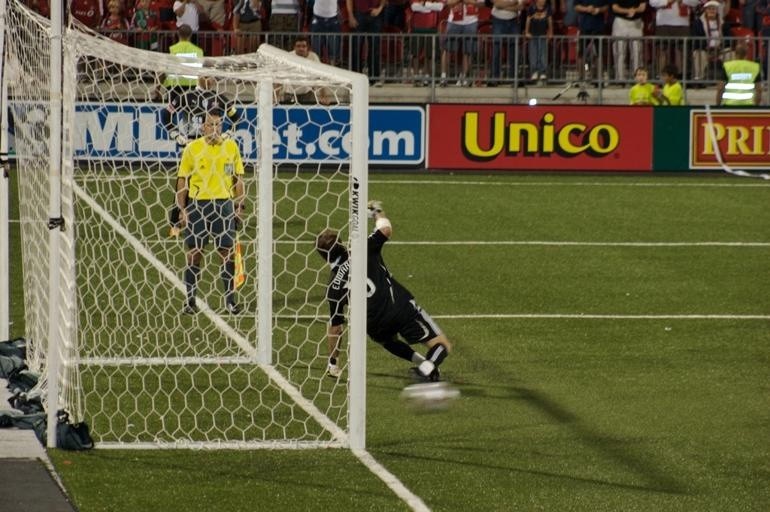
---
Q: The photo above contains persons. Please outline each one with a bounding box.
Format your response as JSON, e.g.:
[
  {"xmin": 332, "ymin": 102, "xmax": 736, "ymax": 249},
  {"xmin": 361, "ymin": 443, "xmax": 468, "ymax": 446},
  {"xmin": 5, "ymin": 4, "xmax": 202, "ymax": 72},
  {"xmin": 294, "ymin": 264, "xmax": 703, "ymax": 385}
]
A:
[
  {"xmin": 629, "ymin": 67, "xmax": 660, "ymax": 106},
  {"xmin": 650, "ymin": 64, "xmax": 686, "ymax": 105},
  {"xmin": 565, "ymin": 0, "xmax": 770, "ymax": 89},
  {"xmin": 316, "ymin": 198, "xmax": 454, "ymax": 384},
  {"xmin": 171, "ymin": 108, "xmax": 246, "ymax": 316},
  {"xmin": 716, "ymin": 44, "xmax": 762, "ymax": 106},
  {"xmin": 15, "ymin": 1, "xmax": 554, "ymax": 112}
]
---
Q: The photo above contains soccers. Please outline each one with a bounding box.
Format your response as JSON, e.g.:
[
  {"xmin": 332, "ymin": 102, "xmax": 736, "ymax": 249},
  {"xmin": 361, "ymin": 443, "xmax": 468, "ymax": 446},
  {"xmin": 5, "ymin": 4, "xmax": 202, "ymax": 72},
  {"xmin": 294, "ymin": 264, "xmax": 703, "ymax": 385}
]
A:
[{"xmin": 403, "ymin": 381, "xmax": 459, "ymax": 412}]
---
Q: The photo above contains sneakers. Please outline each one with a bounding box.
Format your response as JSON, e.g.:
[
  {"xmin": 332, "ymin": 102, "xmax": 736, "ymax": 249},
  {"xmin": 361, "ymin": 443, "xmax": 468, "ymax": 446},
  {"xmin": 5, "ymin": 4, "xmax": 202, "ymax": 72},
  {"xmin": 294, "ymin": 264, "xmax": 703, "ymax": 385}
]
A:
[
  {"xmin": 563, "ymin": 70, "xmax": 637, "ymax": 88},
  {"xmin": 408, "ymin": 367, "xmax": 426, "ymax": 385},
  {"xmin": 226, "ymin": 302, "xmax": 240, "ymax": 314},
  {"xmin": 362, "ymin": 67, "xmax": 547, "ymax": 87},
  {"xmin": 184, "ymin": 300, "xmax": 196, "ymax": 313},
  {"xmin": 425, "ymin": 366, "xmax": 442, "ymax": 382}
]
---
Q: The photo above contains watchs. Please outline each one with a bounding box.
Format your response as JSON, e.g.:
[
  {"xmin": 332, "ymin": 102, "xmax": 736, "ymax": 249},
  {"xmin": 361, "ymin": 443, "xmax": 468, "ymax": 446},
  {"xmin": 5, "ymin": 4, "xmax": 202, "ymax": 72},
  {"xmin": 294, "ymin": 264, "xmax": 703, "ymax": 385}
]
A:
[{"xmin": 234, "ymin": 203, "xmax": 245, "ymax": 210}]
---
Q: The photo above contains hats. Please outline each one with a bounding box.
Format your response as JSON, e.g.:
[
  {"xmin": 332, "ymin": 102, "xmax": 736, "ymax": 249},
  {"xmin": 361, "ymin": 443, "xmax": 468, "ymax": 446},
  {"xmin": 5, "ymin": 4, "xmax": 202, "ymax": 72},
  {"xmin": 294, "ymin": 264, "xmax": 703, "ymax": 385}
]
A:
[{"xmin": 704, "ymin": 1, "xmax": 722, "ymax": 9}]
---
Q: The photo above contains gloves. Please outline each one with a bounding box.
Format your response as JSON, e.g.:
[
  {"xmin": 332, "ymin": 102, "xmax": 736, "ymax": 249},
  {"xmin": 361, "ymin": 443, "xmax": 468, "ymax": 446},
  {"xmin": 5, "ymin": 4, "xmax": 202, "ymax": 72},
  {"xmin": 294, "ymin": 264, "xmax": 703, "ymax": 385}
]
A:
[
  {"xmin": 329, "ymin": 357, "xmax": 341, "ymax": 376},
  {"xmin": 170, "ymin": 130, "xmax": 187, "ymax": 147},
  {"xmin": 368, "ymin": 200, "xmax": 382, "ymax": 218}
]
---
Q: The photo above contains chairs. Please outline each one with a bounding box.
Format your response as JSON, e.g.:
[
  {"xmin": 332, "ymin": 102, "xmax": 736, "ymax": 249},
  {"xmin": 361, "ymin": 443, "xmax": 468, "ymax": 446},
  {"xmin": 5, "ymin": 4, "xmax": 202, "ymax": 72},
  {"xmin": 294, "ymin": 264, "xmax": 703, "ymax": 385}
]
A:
[{"xmin": 1, "ymin": 0, "xmax": 770, "ymax": 93}]
